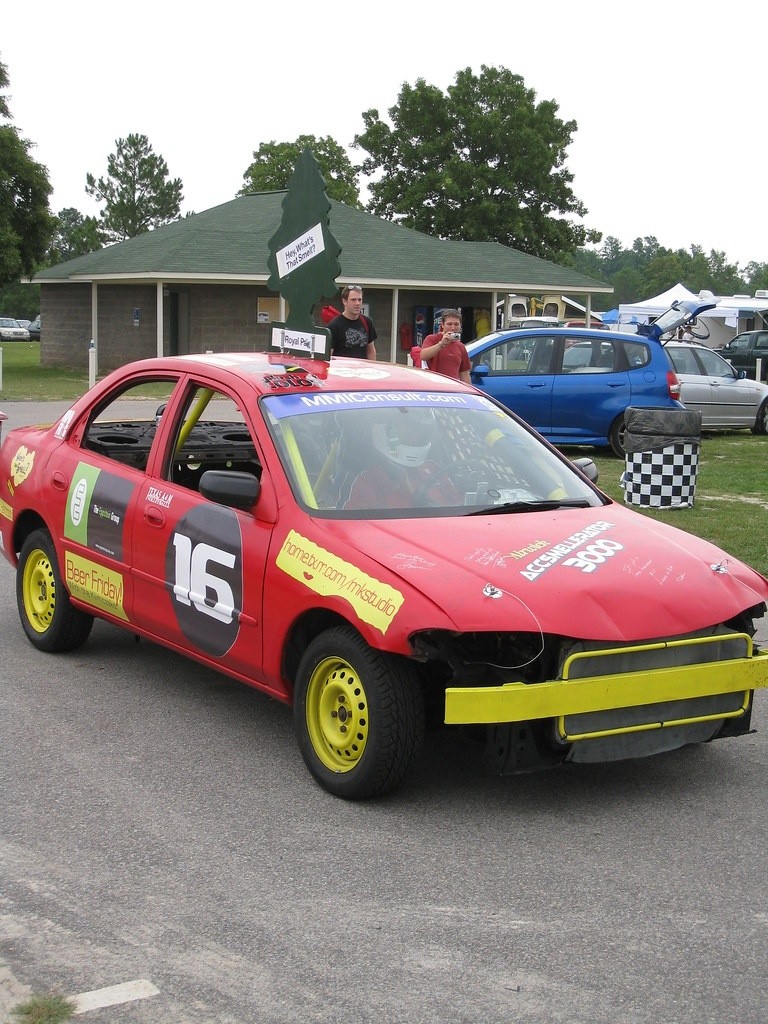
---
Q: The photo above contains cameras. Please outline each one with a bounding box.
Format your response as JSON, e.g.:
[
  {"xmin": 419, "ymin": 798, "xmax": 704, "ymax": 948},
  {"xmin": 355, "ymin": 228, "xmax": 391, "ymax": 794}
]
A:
[{"xmin": 450, "ymin": 333, "xmax": 461, "ymax": 340}]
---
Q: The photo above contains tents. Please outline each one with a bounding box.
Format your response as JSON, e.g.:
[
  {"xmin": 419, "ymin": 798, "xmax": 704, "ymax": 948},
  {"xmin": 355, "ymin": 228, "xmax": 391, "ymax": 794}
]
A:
[{"xmin": 592, "ymin": 283, "xmax": 738, "ymax": 351}]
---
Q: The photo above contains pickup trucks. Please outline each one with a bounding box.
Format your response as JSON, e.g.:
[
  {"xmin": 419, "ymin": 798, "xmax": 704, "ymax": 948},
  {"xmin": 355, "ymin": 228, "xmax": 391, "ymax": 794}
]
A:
[{"xmin": 711, "ymin": 330, "xmax": 768, "ymax": 381}]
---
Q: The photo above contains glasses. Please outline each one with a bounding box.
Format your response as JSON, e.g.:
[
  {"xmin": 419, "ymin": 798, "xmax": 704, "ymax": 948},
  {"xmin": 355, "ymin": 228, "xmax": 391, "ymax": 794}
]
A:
[{"xmin": 345, "ymin": 285, "xmax": 362, "ymax": 291}]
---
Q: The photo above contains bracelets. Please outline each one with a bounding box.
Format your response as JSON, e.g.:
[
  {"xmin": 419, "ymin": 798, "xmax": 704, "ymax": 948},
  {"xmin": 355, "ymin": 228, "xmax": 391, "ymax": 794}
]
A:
[{"xmin": 439, "ymin": 341, "xmax": 447, "ymax": 348}]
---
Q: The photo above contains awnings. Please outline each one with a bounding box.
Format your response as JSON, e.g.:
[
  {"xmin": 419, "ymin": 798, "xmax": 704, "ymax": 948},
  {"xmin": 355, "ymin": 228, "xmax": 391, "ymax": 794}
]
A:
[{"xmin": 729, "ymin": 307, "xmax": 768, "ymax": 319}]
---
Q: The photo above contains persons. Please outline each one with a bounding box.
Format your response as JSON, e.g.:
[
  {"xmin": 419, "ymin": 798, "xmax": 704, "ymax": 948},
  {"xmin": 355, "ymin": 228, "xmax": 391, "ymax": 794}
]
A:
[
  {"xmin": 337, "ymin": 407, "xmax": 462, "ymax": 510},
  {"xmin": 326, "ymin": 285, "xmax": 378, "ymax": 361},
  {"xmin": 420, "ymin": 309, "xmax": 472, "ymax": 384}
]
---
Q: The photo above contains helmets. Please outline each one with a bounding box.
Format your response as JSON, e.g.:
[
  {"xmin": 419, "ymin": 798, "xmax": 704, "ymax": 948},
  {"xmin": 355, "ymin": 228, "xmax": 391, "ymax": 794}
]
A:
[{"xmin": 371, "ymin": 406, "xmax": 437, "ymax": 466}]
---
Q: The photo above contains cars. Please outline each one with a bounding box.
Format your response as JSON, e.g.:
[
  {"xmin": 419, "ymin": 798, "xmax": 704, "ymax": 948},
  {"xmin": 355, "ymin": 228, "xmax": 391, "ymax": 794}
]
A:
[
  {"xmin": 561, "ymin": 339, "xmax": 768, "ymax": 437},
  {"xmin": 0, "ymin": 318, "xmax": 31, "ymax": 343},
  {"xmin": 17, "ymin": 315, "xmax": 40, "ymax": 342},
  {"xmin": 463, "ymin": 324, "xmax": 687, "ymax": 461},
  {"xmin": 0, "ymin": 353, "xmax": 768, "ymax": 799},
  {"xmin": 501, "ymin": 296, "xmax": 716, "ymax": 347}
]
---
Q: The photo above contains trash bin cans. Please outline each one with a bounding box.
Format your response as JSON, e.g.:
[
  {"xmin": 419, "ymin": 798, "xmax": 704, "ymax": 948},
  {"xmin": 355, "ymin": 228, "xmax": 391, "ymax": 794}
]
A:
[{"xmin": 622, "ymin": 406, "xmax": 702, "ymax": 512}]
